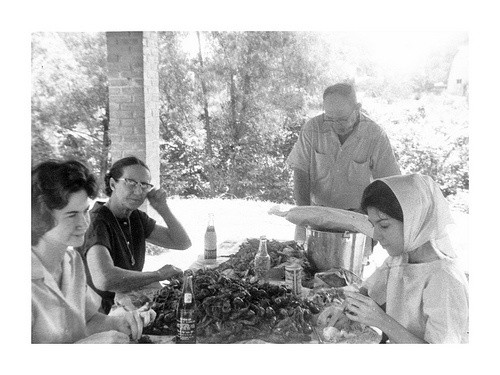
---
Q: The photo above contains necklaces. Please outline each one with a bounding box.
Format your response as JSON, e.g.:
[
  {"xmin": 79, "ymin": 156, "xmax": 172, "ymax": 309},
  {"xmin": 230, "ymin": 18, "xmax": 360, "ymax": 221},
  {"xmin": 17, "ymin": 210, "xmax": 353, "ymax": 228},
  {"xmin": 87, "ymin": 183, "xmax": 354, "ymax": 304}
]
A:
[{"xmin": 108, "ymin": 198, "xmax": 135, "ymax": 266}]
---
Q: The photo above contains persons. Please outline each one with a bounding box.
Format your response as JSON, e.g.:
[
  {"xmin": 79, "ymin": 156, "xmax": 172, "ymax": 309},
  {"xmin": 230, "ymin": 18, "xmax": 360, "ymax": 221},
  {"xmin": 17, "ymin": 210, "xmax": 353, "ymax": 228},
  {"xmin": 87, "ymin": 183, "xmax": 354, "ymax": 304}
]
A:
[
  {"xmin": 287, "ymin": 83, "xmax": 402, "ymax": 242},
  {"xmin": 73, "ymin": 157, "xmax": 192, "ymax": 315},
  {"xmin": 31, "ymin": 160, "xmax": 156, "ymax": 344},
  {"xmin": 344, "ymin": 174, "xmax": 469, "ymax": 344}
]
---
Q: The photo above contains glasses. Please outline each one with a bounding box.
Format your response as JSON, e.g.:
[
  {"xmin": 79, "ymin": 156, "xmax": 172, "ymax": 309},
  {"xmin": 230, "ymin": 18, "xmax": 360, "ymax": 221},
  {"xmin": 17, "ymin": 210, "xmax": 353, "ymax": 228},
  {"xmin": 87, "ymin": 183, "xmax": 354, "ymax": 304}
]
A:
[
  {"xmin": 323, "ymin": 106, "xmax": 356, "ymax": 123},
  {"xmin": 117, "ymin": 178, "xmax": 154, "ymax": 192}
]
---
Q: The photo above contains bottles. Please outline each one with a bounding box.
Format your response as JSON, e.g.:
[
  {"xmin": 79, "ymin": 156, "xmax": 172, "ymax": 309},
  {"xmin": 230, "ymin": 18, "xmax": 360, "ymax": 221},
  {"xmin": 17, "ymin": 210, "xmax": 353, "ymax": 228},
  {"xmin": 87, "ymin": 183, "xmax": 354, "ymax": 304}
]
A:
[
  {"xmin": 285, "ymin": 257, "xmax": 302, "ymax": 297},
  {"xmin": 176, "ymin": 269, "xmax": 196, "ymax": 344},
  {"xmin": 204, "ymin": 212, "xmax": 216, "ymax": 266},
  {"xmin": 254, "ymin": 236, "xmax": 270, "ymax": 281}
]
